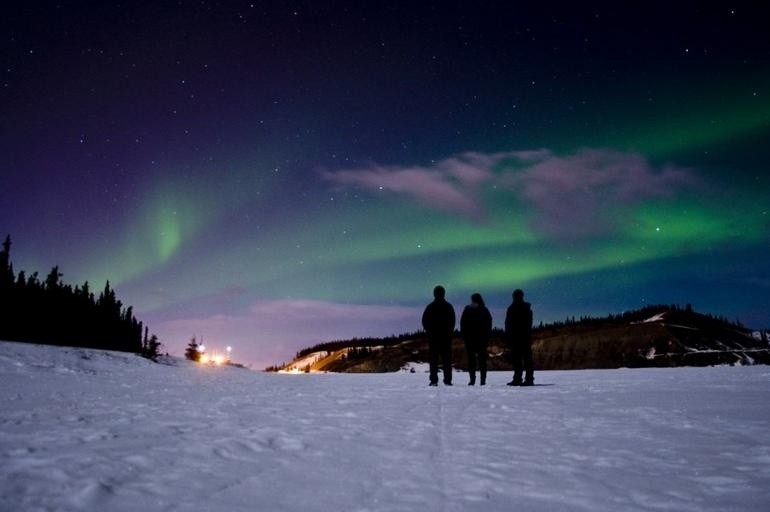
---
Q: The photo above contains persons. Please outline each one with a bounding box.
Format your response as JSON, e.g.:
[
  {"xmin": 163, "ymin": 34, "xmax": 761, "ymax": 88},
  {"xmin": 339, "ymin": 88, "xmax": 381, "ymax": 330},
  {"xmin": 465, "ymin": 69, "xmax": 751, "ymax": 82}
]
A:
[
  {"xmin": 422, "ymin": 285, "xmax": 456, "ymax": 387},
  {"xmin": 505, "ymin": 288, "xmax": 536, "ymax": 386},
  {"xmin": 460, "ymin": 292, "xmax": 493, "ymax": 386}
]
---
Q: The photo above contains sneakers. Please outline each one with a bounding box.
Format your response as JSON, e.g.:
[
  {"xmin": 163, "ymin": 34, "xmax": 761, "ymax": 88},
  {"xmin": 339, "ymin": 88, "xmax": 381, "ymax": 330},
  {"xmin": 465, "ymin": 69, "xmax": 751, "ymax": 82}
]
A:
[
  {"xmin": 443, "ymin": 379, "xmax": 454, "ymax": 386},
  {"xmin": 429, "ymin": 380, "xmax": 438, "ymax": 386},
  {"xmin": 467, "ymin": 376, "xmax": 487, "ymax": 386},
  {"xmin": 506, "ymin": 376, "xmax": 536, "ymax": 387}
]
